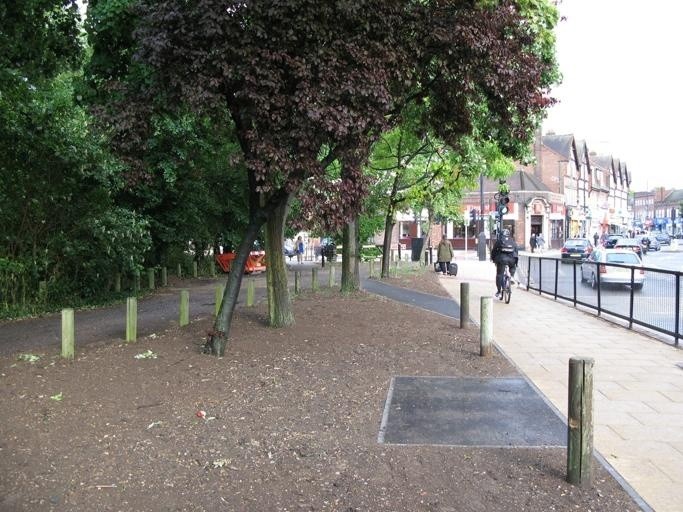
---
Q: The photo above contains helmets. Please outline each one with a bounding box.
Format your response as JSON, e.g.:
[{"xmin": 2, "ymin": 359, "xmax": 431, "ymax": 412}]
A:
[{"xmin": 501, "ymin": 229, "xmax": 510, "ymax": 236}]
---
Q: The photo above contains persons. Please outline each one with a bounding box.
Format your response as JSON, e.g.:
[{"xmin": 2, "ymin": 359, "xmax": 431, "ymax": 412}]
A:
[
  {"xmin": 573, "ymin": 231, "xmax": 610, "ymax": 247},
  {"xmin": 420, "ymin": 232, "xmax": 426, "ymax": 237},
  {"xmin": 403, "ymin": 233, "xmax": 413, "ymax": 239},
  {"xmin": 295, "ymin": 236, "xmax": 305, "ymax": 264},
  {"xmin": 530, "ymin": 232, "xmax": 546, "ymax": 254},
  {"xmin": 437, "ymin": 235, "xmax": 454, "ymax": 274},
  {"xmin": 490, "ymin": 228, "xmax": 519, "ymax": 297},
  {"xmin": 620, "ymin": 229, "xmax": 647, "ymax": 238}
]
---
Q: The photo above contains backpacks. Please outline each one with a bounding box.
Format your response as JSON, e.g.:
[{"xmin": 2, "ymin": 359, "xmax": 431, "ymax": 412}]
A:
[{"xmin": 500, "ymin": 237, "xmax": 514, "ymax": 252}]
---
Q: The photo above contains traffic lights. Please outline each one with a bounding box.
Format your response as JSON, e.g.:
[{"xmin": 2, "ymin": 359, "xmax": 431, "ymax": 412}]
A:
[
  {"xmin": 498, "ymin": 188, "xmax": 510, "ymax": 216},
  {"xmin": 470, "ymin": 209, "xmax": 476, "ymax": 224}
]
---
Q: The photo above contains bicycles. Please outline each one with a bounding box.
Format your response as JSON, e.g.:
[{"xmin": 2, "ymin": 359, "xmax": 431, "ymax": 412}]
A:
[{"xmin": 490, "ymin": 255, "xmax": 512, "ymax": 304}]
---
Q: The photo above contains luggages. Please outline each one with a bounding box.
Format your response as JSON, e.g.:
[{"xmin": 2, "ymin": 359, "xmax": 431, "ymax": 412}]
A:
[{"xmin": 449, "ymin": 263, "xmax": 458, "ymax": 276}]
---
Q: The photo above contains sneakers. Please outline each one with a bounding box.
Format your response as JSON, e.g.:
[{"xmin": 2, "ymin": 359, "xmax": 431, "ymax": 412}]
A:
[
  {"xmin": 495, "ymin": 290, "xmax": 501, "ymax": 297},
  {"xmin": 510, "ymin": 277, "xmax": 515, "ymax": 285}
]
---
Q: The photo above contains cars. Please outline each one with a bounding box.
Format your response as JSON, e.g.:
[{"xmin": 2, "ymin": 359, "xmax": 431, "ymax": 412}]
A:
[
  {"xmin": 283, "ymin": 238, "xmax": 296, "ymax": 256},
  {"xmin": 320, "ymin": 237, "xmax": 335, "ymax": 247},
  {"xmin": 561, "ymin": 230, "xmax": 671, "ymax": 289}
]
